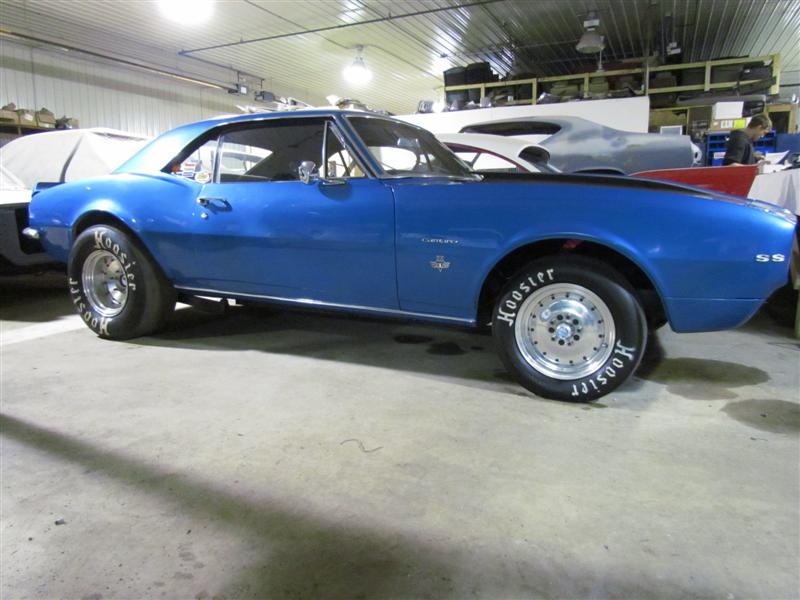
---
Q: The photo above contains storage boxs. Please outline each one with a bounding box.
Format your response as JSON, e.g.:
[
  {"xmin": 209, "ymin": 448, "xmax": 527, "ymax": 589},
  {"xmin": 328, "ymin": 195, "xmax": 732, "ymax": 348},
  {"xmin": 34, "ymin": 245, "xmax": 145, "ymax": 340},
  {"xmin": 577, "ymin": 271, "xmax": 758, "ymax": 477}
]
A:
[
  {"xmin": 0, "ymin": 110, "xmax": 18, "ymax": 123},
  {"xmin": 19, "ymin": 111, "xmax": 38, "ymax": 126},
  {"xmin": 710, "ymin": 121, "xmax": 732, "ymax": 132},
  {"xmin": 734, "ymin": 120, "xmax": 745, "ymax": 129},
  {"xmin": 37, "ymin": 111, "xmax": 55, "ymax": 128},
  {"xmin": 712, "ymin": 101, "xmax": 744, "ymax": 120},
  {"xmin": 57, "ymin": 118, "xmax": 74, "ymax": 130}
]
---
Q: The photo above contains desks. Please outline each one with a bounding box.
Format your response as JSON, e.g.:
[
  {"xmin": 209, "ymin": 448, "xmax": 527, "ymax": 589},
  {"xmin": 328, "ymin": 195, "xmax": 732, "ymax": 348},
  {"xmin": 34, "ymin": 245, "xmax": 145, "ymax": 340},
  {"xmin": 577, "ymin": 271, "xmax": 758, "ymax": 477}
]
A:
[{"xmin": 747, "ymin": 167, "xmax": 800, "ymax": 290}]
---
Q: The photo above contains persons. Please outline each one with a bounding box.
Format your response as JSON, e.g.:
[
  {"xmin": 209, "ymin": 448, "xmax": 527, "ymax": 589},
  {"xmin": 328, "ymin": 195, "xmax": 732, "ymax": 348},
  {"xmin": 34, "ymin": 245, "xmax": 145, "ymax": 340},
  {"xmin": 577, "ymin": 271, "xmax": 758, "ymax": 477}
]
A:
[{"xmin": 722, "ymin": 114, "xmax": 772, "ymax": 168}]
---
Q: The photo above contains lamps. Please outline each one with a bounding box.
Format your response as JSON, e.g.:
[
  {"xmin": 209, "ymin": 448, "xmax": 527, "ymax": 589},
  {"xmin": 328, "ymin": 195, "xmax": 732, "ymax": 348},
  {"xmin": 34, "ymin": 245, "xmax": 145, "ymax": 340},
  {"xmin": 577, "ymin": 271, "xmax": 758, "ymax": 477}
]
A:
[
  {"xmin": 229, "ymin": 71, "xmax": 248, "ymax": 96},
  {"xmin": 256, "ymin": 79, "xmax": 273, "ymax": 103},
  {"xmin": 575, "ymin": 11, "xmax": 604, "ymax": 53},
  {"xmin": 347, "ymin": 45, "xmax": 371, "ymax": 87}
]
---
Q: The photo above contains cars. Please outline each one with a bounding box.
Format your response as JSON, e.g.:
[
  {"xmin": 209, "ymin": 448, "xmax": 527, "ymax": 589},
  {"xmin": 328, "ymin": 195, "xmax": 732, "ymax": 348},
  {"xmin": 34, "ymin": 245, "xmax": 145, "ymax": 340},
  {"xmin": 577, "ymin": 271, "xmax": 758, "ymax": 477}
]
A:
[{"xmin": 0, "ymin": 104, "xmax": 800, "ymax": 404}]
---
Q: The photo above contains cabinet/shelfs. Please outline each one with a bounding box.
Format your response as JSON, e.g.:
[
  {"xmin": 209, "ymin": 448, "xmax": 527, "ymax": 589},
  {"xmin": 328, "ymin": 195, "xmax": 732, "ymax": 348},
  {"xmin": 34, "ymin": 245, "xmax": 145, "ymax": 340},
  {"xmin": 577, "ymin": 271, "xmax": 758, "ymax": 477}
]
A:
[{"xmin": 439, "ymin": 53, "xmax": 797, "ymax": 167}]
